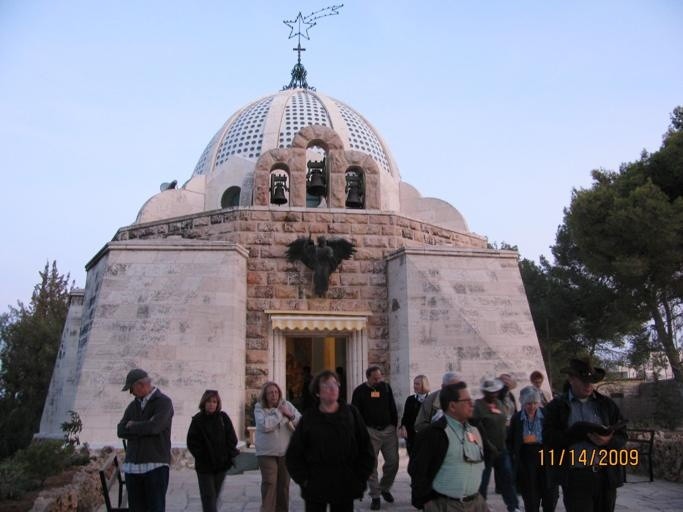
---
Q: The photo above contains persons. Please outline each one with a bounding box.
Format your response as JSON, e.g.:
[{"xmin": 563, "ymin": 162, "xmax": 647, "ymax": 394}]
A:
[
  {"xmin": 186, "ymin": 390, "xmax": 240, "ymax": 512},
  {"xmin": 254, "ymin": 366, "xmax": 399, "ymax": 511},
  {"xmin": 312, "ymin": 235, "xmax": 334, "ymax": 297},
  {"xmin": 399, "ymin": 371, "xmax": 552, "ymax": 511},
  {"xmin": 542, "ymin": 359, "xmax": 627, "ymax": 511},
  {"xmin": 117, "ymin": 369, "xmax": 173, "ymax": 511}
]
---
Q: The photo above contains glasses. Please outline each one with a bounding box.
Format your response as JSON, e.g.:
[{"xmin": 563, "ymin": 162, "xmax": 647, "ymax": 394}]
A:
[
  {"xmin": 455, "ymin": 397, "xmax": 472, "ymax": 403},
  {"xmin": 318, "ymin": 380, "xmax": 340, "ymax": 389}
]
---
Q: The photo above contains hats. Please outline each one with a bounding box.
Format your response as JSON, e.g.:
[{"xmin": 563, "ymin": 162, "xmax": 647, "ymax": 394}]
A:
[
  {"xmin": 479, "ymin": 374, "xmax": 505, "ymax": 393},
  {"xmin": 496, "ymin": 374, "xmax": 517, "ymax": 391},
  {"xmin": 559, "ymin": 355, "xmax": 606, "ymax": 384},
  {"xmin": 121, "ymin": 368, "xmax": 148, "ymax": 392}
]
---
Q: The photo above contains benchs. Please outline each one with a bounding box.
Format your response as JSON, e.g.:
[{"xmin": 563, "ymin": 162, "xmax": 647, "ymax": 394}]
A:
[
  {"xmin": 98, "ymin": 453, "xmax": 130, "ymax": 510},
  {"xmin": 621, "ymin": 428, "xmax": 656, "ymax": 482}
]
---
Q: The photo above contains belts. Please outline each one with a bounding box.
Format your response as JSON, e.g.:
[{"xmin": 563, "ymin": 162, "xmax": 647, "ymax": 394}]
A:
[{"xmin": 586, "ymin": 466, "xmax": 603, "ymax": 472}]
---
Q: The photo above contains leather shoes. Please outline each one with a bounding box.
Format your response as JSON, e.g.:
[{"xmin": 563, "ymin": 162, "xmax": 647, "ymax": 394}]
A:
[
  {"xmin": 370, "ymin": 497, "xmax": 381, "ymax": 511},
  {"xmin": 440, "ymin": 493, "xmax": 479, "ymax": 502},
  {"xmin": 381, "ymin": 489, "xmax": 395, "ymax": 504}
]
